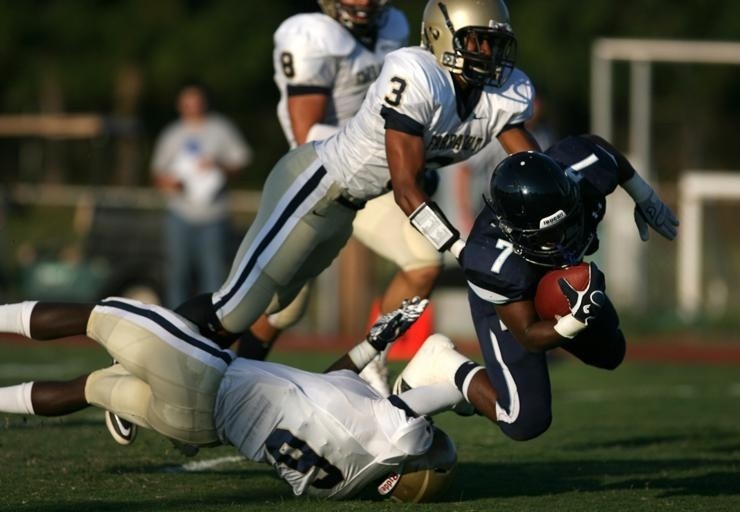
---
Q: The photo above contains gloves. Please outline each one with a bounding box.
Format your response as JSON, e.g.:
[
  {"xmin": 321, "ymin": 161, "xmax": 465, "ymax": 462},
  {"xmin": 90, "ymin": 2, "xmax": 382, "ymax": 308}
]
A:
[
  {"xmin": 633, "ymin": 188, "xmax": 681, "ymax": 244},
  {"xmin": 553, "ymin": 260, "xmax": 606, "ymax": 340},
  {"xmin": 346, "ymin": 295, "xmax": 431, "ymax": 372}
]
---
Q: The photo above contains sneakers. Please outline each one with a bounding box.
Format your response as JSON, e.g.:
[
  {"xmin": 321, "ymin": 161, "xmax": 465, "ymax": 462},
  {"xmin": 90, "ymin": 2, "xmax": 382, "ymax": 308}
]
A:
[
  {"xmin": 389, "ymin": 333, "xmax": 456, "ymax": 398},
  {"xmin": 104, "ymin": 358, "xmax": 140, "ymax": 446},
  {"xmin": 359, "ymin": 357, "xmax": 390, "ymax": 402}
]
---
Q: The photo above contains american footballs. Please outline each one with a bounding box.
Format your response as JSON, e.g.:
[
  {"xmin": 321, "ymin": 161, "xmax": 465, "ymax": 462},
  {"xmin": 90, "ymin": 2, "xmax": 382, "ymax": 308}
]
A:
[{"xmin": 535, "ymin": 265, "xmax": 590, "ymax": 321}]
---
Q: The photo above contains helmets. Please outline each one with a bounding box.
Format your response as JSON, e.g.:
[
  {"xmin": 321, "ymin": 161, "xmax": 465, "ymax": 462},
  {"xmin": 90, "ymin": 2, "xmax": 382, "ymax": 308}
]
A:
[
  {"xmin": 418, "ymin": 0, "xmax": 513, "ymax": 87},
  {"xmin": 368, "ymin": 424, "xmax": 458, "ymax": 503},
  {"xmin": 490, "ymin": 148, "xmax": 581, "ymax": 265},
  {"xmin": 316, "ymin": 0, "xmax": 394, "ymax": 38}
]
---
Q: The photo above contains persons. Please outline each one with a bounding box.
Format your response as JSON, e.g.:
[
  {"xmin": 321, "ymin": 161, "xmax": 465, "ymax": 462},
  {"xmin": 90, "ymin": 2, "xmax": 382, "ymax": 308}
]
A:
[
  {"xmin": 172, "ymin": 0, "xmax": 543, "ymax": 361},
  {"xmin": 433, "ymin": 76, "xmax": 559, "ymax": 287},
  {"xmin": 0, "ymin": 297, "xmax": 478, "ymax": 504},
  {"xmin": 390, "ymin": 133, "xmax": 680, "ymax": 440},
  {"xmin": 143, "ymin": 89, "xmax": 255, "ymax": 309},
  {"xmin": 236, "ymin": 1, "xmax": 441, "ymax": 398}
]
